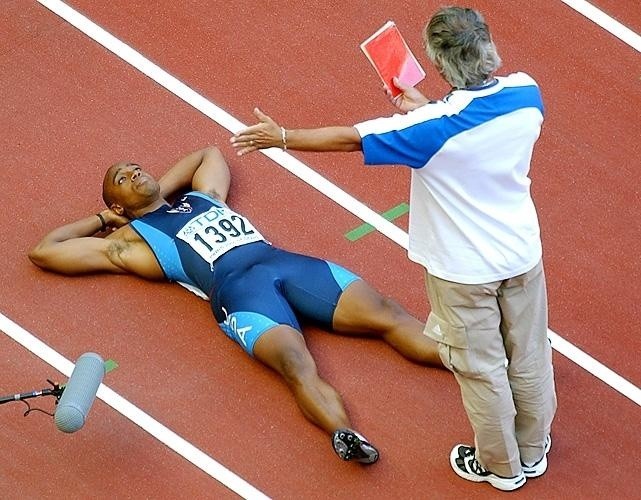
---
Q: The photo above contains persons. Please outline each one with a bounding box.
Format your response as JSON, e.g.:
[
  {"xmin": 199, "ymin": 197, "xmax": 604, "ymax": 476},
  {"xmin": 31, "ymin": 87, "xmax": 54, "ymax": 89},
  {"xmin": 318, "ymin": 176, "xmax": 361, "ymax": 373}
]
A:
[
  {"xmin": 27, "ymin": 146, "xmax": 441, "ymax": 463},
  {"xmin": 229, "ymin": 6, "xmax": 557, "ymax": 492}
]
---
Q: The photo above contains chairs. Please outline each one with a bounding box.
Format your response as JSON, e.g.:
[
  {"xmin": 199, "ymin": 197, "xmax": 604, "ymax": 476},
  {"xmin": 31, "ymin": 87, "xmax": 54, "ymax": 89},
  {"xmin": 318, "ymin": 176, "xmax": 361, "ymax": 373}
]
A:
[
  {"xmin": 331, "ymin": 427, "xmax": 380, "ymax": 464},
  {"xmin": 450, "ymin": 434, "xmax": 551, "ymax": 491}
]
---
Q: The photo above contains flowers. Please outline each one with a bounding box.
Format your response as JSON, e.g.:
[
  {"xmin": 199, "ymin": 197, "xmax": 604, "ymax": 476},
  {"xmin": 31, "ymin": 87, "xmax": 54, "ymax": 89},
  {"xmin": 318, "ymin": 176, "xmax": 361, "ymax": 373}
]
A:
[{"xmin": 53, "ymin": 351, "xmax": 106, "ymax": 435}]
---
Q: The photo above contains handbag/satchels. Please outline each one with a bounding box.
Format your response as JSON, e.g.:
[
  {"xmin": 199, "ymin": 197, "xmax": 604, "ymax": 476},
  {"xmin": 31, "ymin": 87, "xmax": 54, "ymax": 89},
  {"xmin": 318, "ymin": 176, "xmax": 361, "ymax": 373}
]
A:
[
  {"xmin": 96, "ymin": 214, "xmax": 106, "ymax": 232},
  {"xmin": 282, "ymin": 127, "xmax": 286, "ymax": 152}
]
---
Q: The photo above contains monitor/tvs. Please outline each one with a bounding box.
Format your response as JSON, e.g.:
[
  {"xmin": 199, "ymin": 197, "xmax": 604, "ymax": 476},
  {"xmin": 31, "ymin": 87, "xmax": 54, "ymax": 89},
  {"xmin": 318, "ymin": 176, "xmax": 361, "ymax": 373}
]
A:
[{"xmin": 359, "ymin": 21, "xmax": 427, "ymax": 100}]
